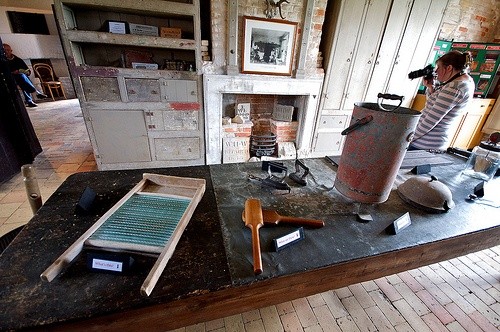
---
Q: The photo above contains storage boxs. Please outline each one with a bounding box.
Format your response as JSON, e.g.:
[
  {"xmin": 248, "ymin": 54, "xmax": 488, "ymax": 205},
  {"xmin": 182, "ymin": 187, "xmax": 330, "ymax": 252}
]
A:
[
  {"xmin": 161, "ymin": 26, "xmax": 183, "ymax": 39},
  {"xmin": 129, "ymin": 23, "xmax": 159, "ymax": 37},
  {"xmin": 109, "ymin": 21, "xmax": 126, "ymax": 34}
]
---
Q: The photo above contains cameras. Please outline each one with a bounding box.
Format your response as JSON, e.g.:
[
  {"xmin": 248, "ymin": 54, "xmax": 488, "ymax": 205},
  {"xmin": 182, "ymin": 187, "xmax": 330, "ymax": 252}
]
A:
[{"xmin": 408, "ymin": 64, "xmax": 434, "ymax": 80}]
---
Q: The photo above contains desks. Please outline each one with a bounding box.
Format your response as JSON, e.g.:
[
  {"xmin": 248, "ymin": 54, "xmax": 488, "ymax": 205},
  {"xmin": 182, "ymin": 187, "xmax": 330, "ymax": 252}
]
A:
[{"xmin": 0, "ymin": 148, "xmax": 500, "ymax": 332}]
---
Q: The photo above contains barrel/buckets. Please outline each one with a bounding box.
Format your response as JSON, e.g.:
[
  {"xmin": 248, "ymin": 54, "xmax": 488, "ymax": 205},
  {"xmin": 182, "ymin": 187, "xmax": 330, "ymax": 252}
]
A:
[{"xmin": 335, "ymin": 103, "xmax": 422, "ymax": 203}]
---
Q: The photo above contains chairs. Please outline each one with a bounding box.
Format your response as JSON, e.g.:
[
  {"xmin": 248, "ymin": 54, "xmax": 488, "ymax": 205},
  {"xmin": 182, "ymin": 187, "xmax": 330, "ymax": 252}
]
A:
[{"xmin": 33, "ymin": 63, "xmax": 67, "ymax": 102}]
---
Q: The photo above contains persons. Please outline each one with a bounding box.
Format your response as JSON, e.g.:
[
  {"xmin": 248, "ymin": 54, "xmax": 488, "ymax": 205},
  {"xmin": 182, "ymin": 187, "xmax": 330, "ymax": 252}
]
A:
[
  {"xmin": 407, "ymin": 50, "xmax": 475, "ymax": 151},
  {"xmin": 0, "ymin": 44, "xmax": 47, "ymax": 107}
]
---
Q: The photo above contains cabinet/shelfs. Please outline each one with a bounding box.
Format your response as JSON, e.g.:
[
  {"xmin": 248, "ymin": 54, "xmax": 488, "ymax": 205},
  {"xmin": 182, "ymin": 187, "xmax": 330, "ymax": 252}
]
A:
[
  {"xmin": 412, "ymin": 95, "xmax": 495, "ymax": 149},
  {"xmin": 364, "ymin": 0, "xmax": 451, "ymax": 108},
  {"xmin": 312, "ymin": 0, "xmax": 393, "ymax": 157},
  {"xmin": 52, "ymin": 0, "xmax": 205, "ymax": 171}
]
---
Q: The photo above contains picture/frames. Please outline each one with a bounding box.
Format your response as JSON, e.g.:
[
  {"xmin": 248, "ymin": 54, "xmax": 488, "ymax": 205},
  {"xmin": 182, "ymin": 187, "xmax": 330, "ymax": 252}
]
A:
[{"xmin": 240, "ymin": 15, "xmax": 299, "ymax": 76}]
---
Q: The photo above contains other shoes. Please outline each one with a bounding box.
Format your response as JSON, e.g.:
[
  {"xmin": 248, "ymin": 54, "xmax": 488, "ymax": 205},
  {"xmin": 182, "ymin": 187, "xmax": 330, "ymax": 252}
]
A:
[
  {"xmin": 25, "ymin": 101, "xmax": 37, "ymax": 107},
  {"xmin": 36, "ymin": 93, "xmax": 47, "ymax": 98}
]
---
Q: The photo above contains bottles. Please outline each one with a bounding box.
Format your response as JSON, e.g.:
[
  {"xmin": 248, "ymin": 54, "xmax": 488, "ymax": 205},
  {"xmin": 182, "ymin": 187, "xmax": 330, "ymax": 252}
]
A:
[{"xmin": 463, "ymin": 141, "xmax": 500, "ymax": 181}]
